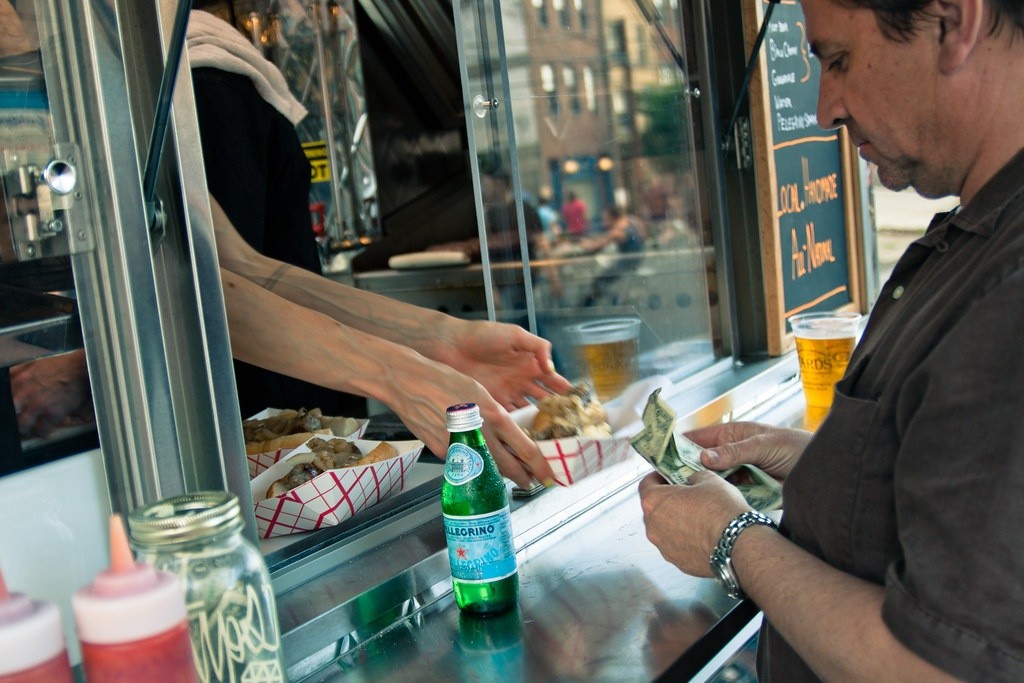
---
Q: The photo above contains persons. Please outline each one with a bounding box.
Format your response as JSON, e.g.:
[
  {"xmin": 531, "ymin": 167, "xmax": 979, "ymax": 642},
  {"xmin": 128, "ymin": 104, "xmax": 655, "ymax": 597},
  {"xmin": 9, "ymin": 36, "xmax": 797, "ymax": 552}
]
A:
[
  {"xmin": 640, "ymin": 0, "xmax": 1024, "ymax": 683},
  {"xmin": 488, "ymin": 169, "xmax": 686, "ymax": 308},
  {"xmin": 0, "ymin": 0, "xmax": 576, "ymax": 491}
]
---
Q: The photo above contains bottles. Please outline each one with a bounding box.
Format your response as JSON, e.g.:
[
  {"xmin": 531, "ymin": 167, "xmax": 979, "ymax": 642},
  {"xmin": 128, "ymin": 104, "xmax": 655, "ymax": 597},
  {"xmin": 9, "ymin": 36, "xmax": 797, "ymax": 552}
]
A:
[
  {"xmin": 442, "ymin": 403, "xmax": 519, "ymax": 620},
  {"xmin": 1, "ymin": 573, "xmax": 73, "ymax": 683},
  {"xmin": 72, "ymin": 515, "xmax": 204, "ymax": 683},
  {"xmin": 129, "ymin": 492, "xmax": 284, "ymax": 683}
]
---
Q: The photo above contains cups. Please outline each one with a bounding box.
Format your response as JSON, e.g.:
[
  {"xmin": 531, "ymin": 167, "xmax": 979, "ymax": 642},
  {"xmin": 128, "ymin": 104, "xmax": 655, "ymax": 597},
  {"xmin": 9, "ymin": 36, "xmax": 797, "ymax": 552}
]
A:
[
  {"xmin": 569, "ymin": 318, "xmax": 639, "ymax": 402},
  {"xmin": 789, "ymin": 312, "xmax": 860, "ymax": 407}
]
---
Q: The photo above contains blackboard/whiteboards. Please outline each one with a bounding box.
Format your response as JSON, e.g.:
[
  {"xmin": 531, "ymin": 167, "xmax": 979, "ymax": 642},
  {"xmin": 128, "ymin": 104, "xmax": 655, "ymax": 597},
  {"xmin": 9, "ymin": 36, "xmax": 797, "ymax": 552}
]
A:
[{"xmin": 741, "ymin": 4, "xmax": 864, "ymax": 356}]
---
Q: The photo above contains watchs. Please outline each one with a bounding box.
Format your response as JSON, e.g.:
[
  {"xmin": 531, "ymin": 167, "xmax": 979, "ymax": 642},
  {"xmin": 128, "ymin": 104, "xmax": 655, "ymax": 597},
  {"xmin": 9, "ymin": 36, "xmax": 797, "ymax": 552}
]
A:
[{"xmin": 709, "ymin": 512, "xmax": 778, "ymax": 598}]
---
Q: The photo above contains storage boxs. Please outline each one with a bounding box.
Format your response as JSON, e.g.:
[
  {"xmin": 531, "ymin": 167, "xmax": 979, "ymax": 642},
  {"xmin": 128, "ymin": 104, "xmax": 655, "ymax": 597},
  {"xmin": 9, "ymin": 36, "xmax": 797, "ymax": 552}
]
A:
[{"xmin": 232, "ymin": 373, "xmax": 676, "ymax": 540}]
